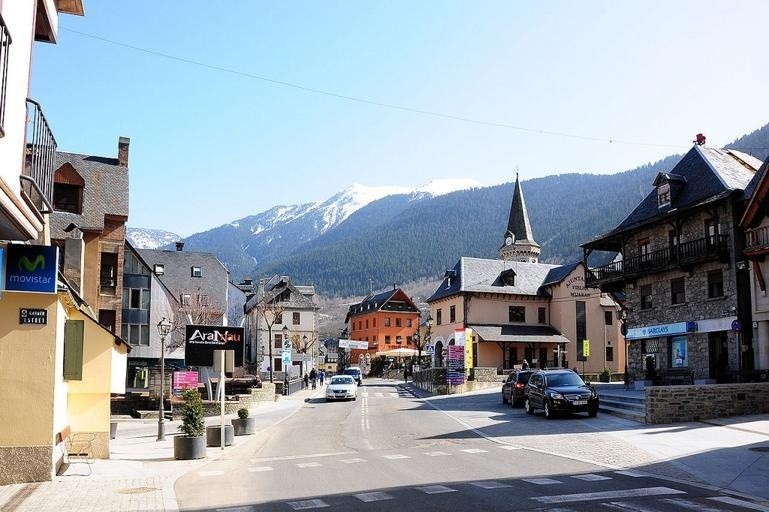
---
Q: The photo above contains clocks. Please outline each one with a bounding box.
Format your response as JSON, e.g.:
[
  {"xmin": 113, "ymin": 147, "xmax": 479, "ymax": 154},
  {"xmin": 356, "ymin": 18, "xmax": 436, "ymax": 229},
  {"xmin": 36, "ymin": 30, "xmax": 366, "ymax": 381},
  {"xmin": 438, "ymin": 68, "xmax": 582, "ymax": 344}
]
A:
[{"xmin": 505, "ymin": 237, "xmax": 513, "ymax": 246}]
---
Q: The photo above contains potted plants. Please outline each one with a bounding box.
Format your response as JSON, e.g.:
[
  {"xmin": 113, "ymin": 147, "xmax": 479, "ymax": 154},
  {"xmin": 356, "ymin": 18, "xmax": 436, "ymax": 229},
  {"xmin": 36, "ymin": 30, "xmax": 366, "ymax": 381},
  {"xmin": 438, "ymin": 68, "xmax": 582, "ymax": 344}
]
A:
[
  {"xmin": 173, "ymin": 386, "xmax": 209, "ymax": 462},
  {"xmin": 604, "ymin": 367, "xmax": 611, "ymax": 382},
  {"xmin": 231, "ymin": 407, "xmax": 257, "ymax": 436}
]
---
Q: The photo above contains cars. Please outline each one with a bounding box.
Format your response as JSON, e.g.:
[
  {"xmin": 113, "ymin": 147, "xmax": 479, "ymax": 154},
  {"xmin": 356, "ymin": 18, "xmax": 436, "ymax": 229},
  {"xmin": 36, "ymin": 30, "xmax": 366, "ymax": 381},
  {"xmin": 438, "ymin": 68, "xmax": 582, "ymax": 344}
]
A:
[
  {"xmin": 324, "ymin": 374, "xmax": 358, "ymax": 402},
  {"xmin": 325, "ymin": 370, "xmax": 335, "ymax": 377}
]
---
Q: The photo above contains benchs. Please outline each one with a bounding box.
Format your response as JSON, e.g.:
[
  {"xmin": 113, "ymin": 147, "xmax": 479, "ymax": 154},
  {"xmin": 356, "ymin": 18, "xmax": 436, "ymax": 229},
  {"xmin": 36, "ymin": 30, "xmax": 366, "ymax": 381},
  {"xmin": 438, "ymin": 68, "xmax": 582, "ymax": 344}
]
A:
[
  {"xmin": 651, "ymin": 366, "xmax": 695, "ymax": 386},
  {"xmin": 58, "ymin": 424, "xmax": 97, "ymax": 477}
]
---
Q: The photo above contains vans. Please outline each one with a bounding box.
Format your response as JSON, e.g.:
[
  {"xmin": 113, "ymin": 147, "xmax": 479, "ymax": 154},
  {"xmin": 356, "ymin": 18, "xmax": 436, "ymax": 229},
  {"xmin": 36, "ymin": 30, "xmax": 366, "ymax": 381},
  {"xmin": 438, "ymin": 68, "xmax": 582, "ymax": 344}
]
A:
[{"xmin": 342, "ymin": 366, "xmax": 362, "ymax": 387}]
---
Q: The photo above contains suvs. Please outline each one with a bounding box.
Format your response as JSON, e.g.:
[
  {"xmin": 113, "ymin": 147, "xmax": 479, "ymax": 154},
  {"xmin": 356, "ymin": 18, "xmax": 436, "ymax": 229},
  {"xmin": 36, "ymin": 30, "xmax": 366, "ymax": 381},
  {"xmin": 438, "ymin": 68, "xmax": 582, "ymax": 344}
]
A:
[
  {"xmin": 502, "ymin": 369, "xmax": 537, "ymax": 410},
  {"xmin": 260, "ymin": 371, "xmax": 278, "ymax": 381},
  {"xmin": 522, "ymin": 365, "xmax": 603, "ymax": 420}
]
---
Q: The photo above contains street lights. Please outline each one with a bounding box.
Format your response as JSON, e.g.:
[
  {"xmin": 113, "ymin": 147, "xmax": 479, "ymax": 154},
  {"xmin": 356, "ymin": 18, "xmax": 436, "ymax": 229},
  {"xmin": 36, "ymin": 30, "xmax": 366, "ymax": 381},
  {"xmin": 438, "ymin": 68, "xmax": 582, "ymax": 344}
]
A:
[
  {"xmin": 396, "ymin": 315, "xmax": 433, "ymax": 364},
  {"xmin": 620, "ymin": 317, "xmax": 630, "ymax": 391},
  {"xmin": 302, "ymin": 334, "xmax": 309, "ymax": 371},
  {"xmin": 154, "ymin": 316, "xmax": 172, "ymax": 442},
  {"xmin": 280, "ymin": 323, "xmax": 291, "ymax": 398}
]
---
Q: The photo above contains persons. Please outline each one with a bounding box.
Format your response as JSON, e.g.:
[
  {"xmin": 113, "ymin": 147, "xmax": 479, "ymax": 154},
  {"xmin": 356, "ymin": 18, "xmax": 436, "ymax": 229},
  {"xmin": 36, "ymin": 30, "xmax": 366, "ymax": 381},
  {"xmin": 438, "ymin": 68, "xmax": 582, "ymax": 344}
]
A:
[
  {"xmin": 522, "ymin": 359, "xmax": 529, "ymax": 370},
  {"xmin": 303, "ymin": 372, "xmax": 308, "ymax": 389},
  {"xmin": 309, "ymin": 368, "xmax": 325, "ymax": 390},
  {"xmin": 403, "ymin": 366, "xmax": 407, "ymax": 384}
]
